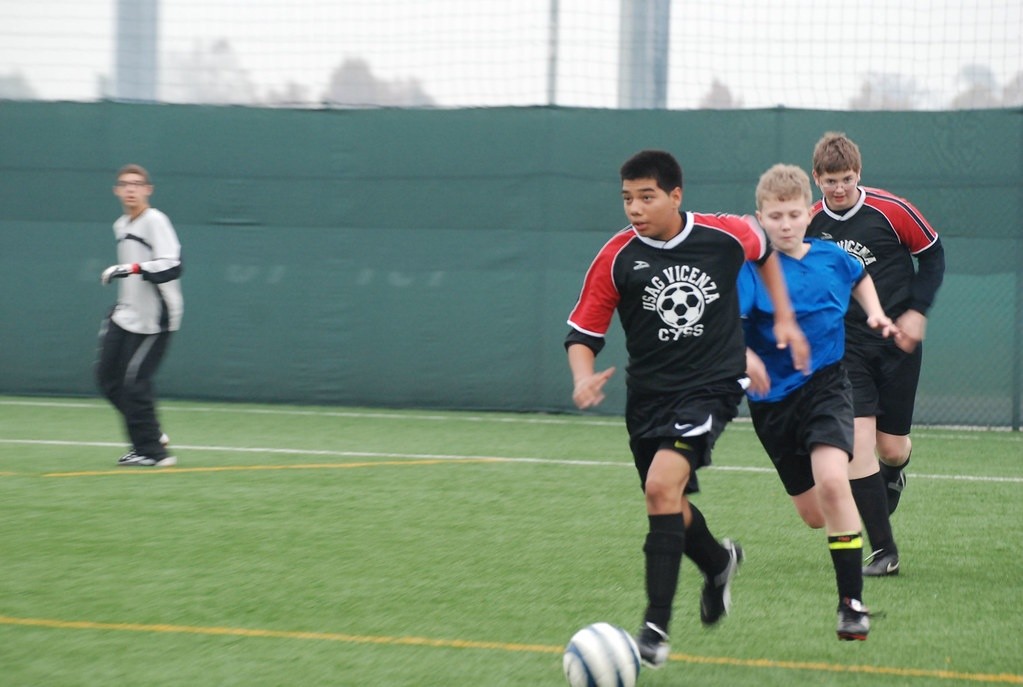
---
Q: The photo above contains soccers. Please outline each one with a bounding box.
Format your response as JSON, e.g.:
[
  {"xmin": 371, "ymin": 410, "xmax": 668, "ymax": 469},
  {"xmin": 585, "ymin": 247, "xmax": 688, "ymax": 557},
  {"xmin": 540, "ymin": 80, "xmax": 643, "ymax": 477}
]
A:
[{"xmin": 563, "ymin": 622, "xmax": 642, "ymax": 687}]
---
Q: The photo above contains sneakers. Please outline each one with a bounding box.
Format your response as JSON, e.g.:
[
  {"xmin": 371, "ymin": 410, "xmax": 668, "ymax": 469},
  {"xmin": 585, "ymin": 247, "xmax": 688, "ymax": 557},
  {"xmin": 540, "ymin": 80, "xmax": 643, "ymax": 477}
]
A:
[
  {"xmin": 861, "ymin": 542, "xmax": 900, "ymax": 576},
  {"xmin": 700, "ymin": 538, "xmax": 745, "ymax": 626},
  {"xmin": 135, "ymin": 456, "xmax": 176, "ymax": 467},
  {"xmin": 117, "ymin": 433, "xmax": 169, "ymax": 464},
  {"xmin": 836, "ymin": 596, "xmax": 869, "ymax": 639},
  {"xmin": 638, "ymin": 621, "xmax": 671, "ymax": 670},
  {"xmin": 884, "ymin": 469, "xmax": 906, "ymax": 514}
]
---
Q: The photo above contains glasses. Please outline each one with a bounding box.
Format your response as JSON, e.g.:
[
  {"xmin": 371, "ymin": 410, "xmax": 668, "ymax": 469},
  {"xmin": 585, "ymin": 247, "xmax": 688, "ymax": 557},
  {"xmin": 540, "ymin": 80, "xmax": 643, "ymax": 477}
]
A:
[{"xmin": 117, "ymin": 180, "xmax": 147, "ymax": 190}]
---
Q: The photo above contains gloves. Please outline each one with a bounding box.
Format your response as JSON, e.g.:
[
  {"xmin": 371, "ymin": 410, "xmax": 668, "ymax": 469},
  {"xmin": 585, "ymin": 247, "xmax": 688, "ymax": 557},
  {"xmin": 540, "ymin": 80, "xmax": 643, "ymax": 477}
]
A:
[{"xmin": 100, "ymin": 262, "xmax": 140, "ymax": 285}]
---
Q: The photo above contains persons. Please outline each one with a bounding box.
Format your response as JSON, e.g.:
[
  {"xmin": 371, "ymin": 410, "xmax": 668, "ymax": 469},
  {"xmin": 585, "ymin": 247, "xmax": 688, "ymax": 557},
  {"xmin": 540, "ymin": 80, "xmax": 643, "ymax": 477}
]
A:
[
  {"xmin": 803, "ymin": 130, "xmax": 946, "ymax": 578},
  {"xmin": 730, "ymin": 164, "xmax": 899, "ymax": 642},
  {"xmin": 562, "ymin": 151, "xmax": 811, "ymax": 669},
  {"xmin": 96, "ymin": 164, "xmax": 185, "ymax": 466}
]
